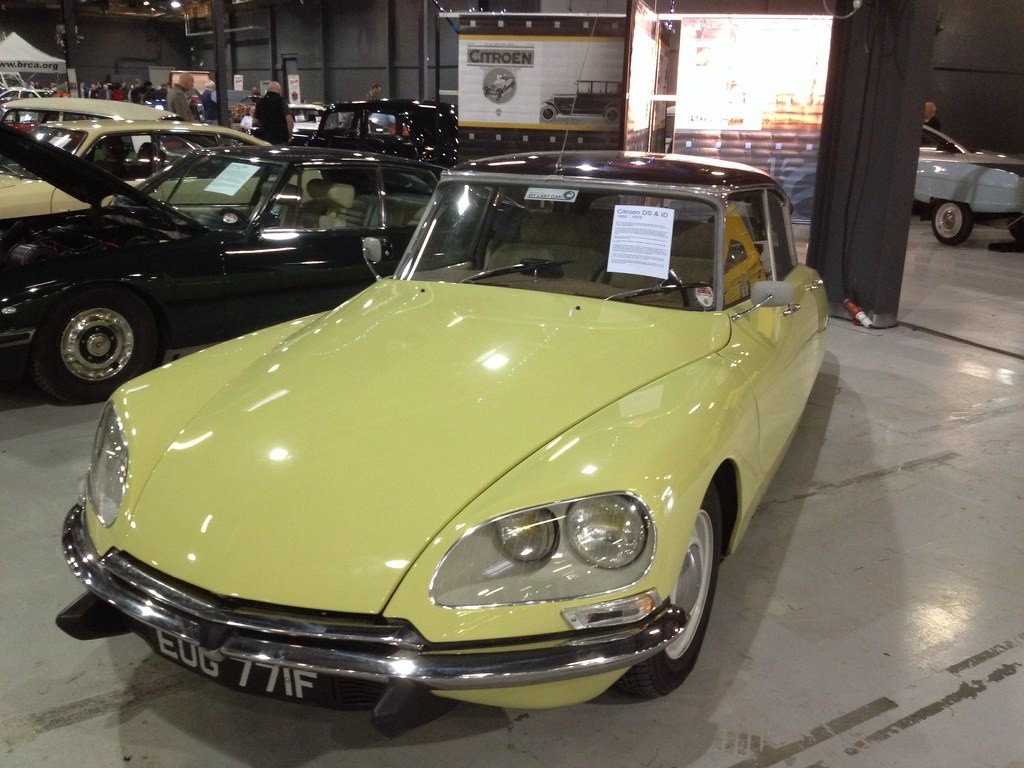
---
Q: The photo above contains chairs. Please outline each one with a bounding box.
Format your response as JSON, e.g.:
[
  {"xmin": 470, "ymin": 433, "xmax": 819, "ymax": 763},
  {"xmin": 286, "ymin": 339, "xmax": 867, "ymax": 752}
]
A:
[
  {"xmin": 86, "ymin": 135, "xmax": 127, "ymax": 172},
  {"xmin": 295, "ymin": 178, "xmax": 354, "ymax": 230},
  {"xmin": 250, "ymin": 181, "xmax": 300, "ymax": 228},
  {"xmin": 483, "ymin": 213, "xmax": 605, "ymax": 286},
  {"xmin": 126, "ymin": 142, "xmax": 166, "ymax": 180},
  {"xmin": 610, "ymin": 223, "xmax": 714, "ymax": 299}
]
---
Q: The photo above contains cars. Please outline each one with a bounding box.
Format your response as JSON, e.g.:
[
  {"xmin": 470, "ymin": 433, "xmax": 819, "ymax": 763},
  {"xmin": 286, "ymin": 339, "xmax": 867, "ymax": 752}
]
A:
[
  {"xmin": 0, "ymin": 121, "xmax": 544, "ymax": 402},
  {"xmin": 919, "ymin": 123, "xmax": 1024, "ymax": 176},
  {"xmin": 288, "ymin": 103, "xmax": 329, "ymax": 145},
  {"xmin": 538, "ymin": 78, "xmax": 624, "ymax": 124},
  {"xmin": 0, "ymin": 87, "xmax": 57, "ymax": 98},
  {"xmin": 914, "ymin": 161, "xmax": 1024, "ymax": 247},
  {"xmin": 313, "ymin": 100, "xmax": 462, "ymax": 170},
  {"xmin": 0, "ymin": 99, "xmax": 185, "ymax": 120},
  {"xmin": 0, "ymin": 121, "xmax": 355, "ymax": 220},
  {"xmin": 55, "ymin": 149, "xmax": 831, "ymax": 737}
]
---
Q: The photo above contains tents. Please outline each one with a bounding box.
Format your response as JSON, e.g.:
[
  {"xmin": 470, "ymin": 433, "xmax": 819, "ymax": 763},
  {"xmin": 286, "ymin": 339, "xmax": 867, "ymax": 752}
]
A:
[{"xmin": 0, "ymin": 32, "xmax": 71, "ymax": 99}]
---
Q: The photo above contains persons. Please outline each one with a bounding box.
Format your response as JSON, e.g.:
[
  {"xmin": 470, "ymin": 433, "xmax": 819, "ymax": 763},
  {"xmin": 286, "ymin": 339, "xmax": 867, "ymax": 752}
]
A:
[
  {"xmin": 253, "ymin": 81, "xmax": 294, "ymax": 145},
  {"xmin": 166, "ymin": 74, "xmax": 196, "ymax": 123},
  {"xmin": 201, "ymin": 80, "xmax": 218, "ymax": 126},
  {"xmin": 920, "ymin": 102, "xmax": 942, "ymax": 147},
  {"xmin": 366, "ymin": 85, "xmax": 381, "ymax": 101},
  {"xmin": 31, "ymin": 79, "xmax": 169, "ymax": 104},
  {"xmin": 241, "ymin": 107, "xmax": 260, "ymax": 133}
]
